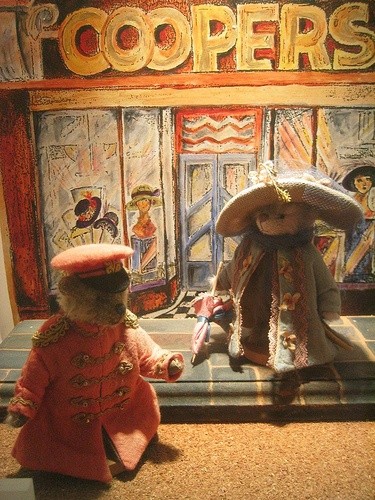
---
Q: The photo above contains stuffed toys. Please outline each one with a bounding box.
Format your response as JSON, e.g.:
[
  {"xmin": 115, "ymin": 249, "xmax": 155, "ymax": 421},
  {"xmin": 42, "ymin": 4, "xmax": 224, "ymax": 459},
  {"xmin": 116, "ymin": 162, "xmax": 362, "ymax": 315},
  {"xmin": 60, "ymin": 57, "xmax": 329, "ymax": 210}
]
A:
[
  {"xmin": 192, "ymin": 160, "xmax": 365, "ymax": 370},
  {"xmin": 6, "ymin": 244, "xmax": 185, "ymax": 482}
]
